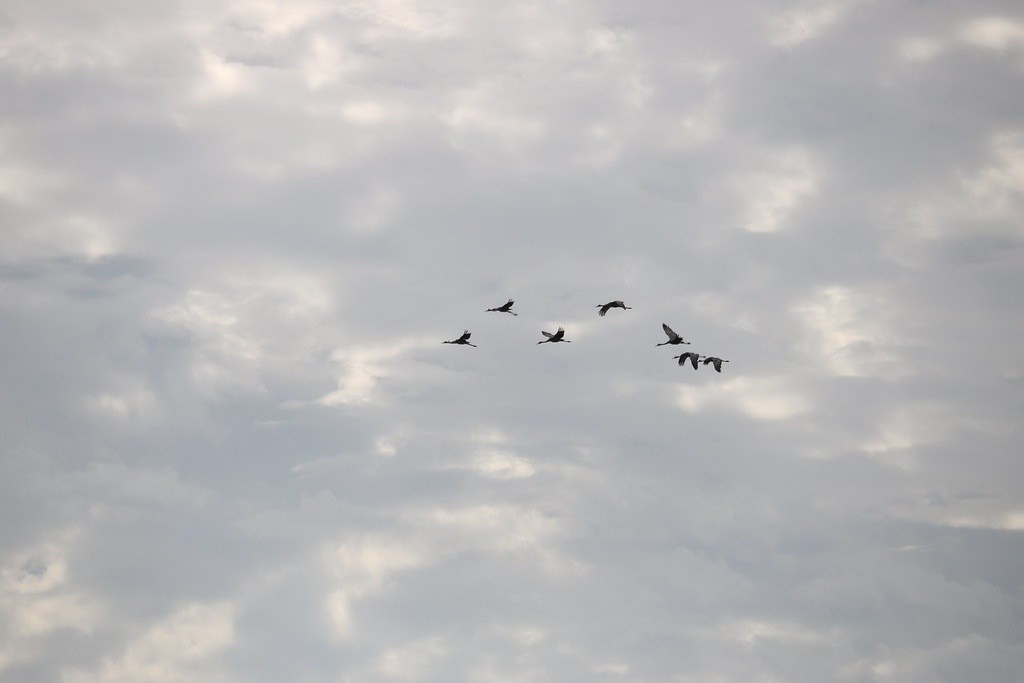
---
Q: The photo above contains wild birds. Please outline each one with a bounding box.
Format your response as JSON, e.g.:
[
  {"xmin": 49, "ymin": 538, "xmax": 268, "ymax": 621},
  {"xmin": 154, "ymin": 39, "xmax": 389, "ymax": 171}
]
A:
[
  {"xmin": 595, "ymin": 299, "xmax": 633, "ymax": 317},
  {"xmin": 484, "ymin": 298, "xmax": 518, "ymax": 317},
  {"xmin": 439, "ymin": 330, "xmax": 478, "ymax": 348},
  {"xmin": 672, "ymin": 351, "xmax": 730, "ymax": 374},
  {"xmin": 535, "ymin": 327, "xmax": 572, "ymax": 346},
  {"xmin": 655, "ymin": 323, "xmax": 692, "ymax": 347}
]
